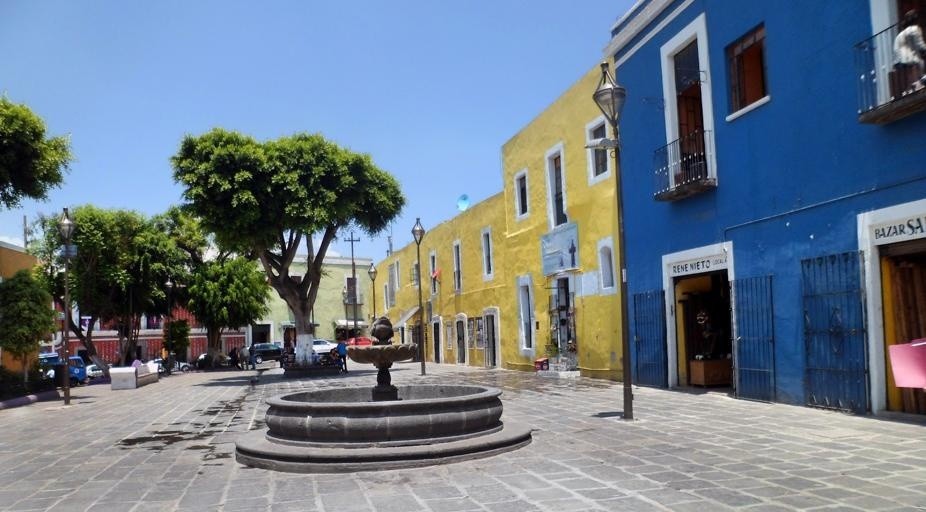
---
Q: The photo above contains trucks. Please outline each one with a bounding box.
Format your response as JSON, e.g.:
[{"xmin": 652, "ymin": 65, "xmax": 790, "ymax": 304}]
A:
[{"xmin": 37, "ymin": 351, "xmax": 86, "ymax": 388}]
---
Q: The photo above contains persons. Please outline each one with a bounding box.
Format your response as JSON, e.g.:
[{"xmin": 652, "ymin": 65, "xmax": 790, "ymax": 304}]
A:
[
  {"xmin": 891, "ymin": 11, "xmax": 925, "ymax": 84},
  {"xmin": 288, "ymin": 337, "xmax": 295, "ymax": 353},
  {"xmin": 230, "ymin": 342, "xmax": 257, "ymax": 369},
  {"xmin": 160, "ymin": 343, "xmax": 171, "ymax": 376},
  {"xmin": 329, "ymin": 338, "xmax": 347, "ymax": 373}
]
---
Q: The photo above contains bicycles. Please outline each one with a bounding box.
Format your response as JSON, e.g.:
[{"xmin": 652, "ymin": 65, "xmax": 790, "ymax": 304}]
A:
[{"xmin": 181, "ymin": 360, "xmax": 200, "ymax": 374}]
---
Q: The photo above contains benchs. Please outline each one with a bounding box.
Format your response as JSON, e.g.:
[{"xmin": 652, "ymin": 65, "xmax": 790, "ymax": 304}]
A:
[
  {"xmin": 280, "ymin": 351, "xmax": 330, "ymax": 367},
  {"xmin": 109, "ymin": 364, "xmax": 160, "ymax": 389}
]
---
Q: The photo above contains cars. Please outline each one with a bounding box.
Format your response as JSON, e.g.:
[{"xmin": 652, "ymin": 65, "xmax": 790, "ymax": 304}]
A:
[
  {"xmin": 142, "ymin": 358, "xmax": 194, "ymax": 374},
  {"xmin": 86, "ymin": 364, "xmax": 112, "ymax": 377},
  {"xmin": 311, "ymin": 338, "xmax": 339, "ymax": 355},
  {"xmin": 344, "ymin": 336, "xmax": 371, "ymax": 345}
]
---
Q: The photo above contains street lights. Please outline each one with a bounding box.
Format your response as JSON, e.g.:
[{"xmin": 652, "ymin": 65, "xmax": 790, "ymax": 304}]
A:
[
  {"xmin": 342, "ymin": 286, "xmax": 348, "ymax": 339},
  {"xmin": 368, "ymin": 262, "xmax": 378, "ymax": 323},
  {"xmin": 591, "ymin": 61, "xmax": 635, "ymax": 419},
  {"xmin": 57, "ymin": 206, "xmax": 74, "ymax": 405},
  {"xmin": 411, "ymin": 217, "xmax": 427, "ymax": 375}
]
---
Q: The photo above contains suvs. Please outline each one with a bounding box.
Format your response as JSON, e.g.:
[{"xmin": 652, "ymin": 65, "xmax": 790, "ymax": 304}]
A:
[{"xmin": 247, "ymin": 343, "xmax": 283, "ymax": 365}]
